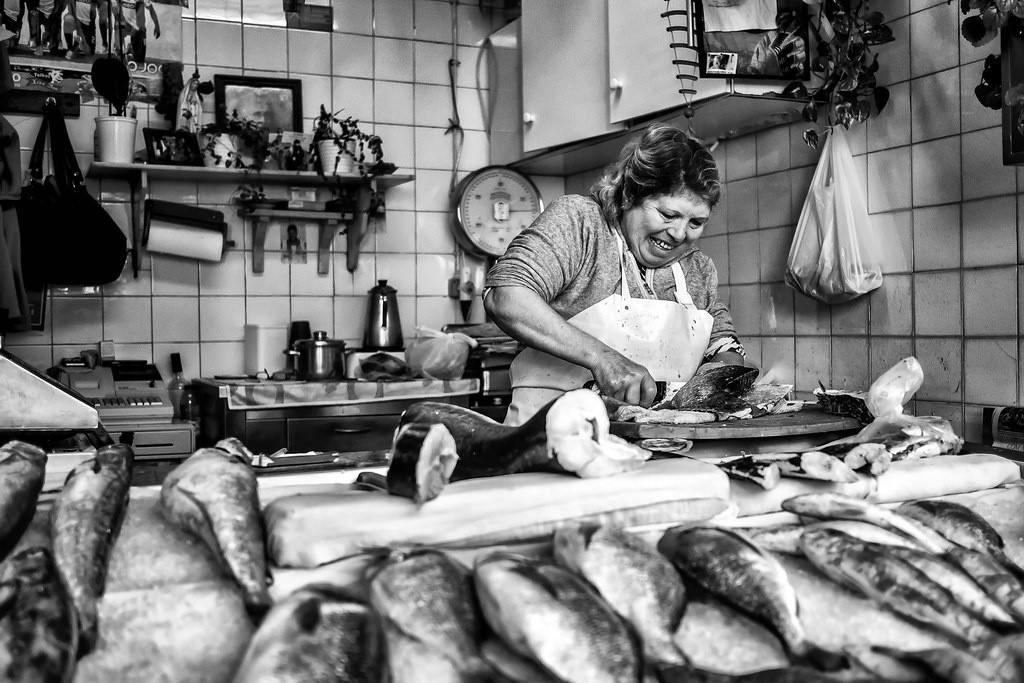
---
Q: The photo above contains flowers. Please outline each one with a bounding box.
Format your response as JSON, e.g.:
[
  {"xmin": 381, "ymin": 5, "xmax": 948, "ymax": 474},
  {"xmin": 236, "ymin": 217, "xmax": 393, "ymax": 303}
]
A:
[
  {"xmin": 307, "ymin": 103, "xmax": 398, "ymax": 216},
  {"xmin": 177, "ymin": 108, "xmax": 283, "ymax": 219}
]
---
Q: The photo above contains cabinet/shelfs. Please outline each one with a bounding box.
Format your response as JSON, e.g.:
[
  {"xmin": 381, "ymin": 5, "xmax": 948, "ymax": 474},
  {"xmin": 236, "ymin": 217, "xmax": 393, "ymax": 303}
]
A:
[
  {"xmin": 191, "ymin": 377, "xmax": 481, "ymax": 455},
  {"xmin": 486, "ymin": 0, "xmax": 825, "ymax": 178}
]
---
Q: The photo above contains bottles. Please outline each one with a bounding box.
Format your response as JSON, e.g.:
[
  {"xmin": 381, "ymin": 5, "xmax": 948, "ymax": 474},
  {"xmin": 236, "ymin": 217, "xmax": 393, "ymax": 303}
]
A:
[{"xmin": 166, "ymin": 352, "xmax": 190, "ymax": 419}]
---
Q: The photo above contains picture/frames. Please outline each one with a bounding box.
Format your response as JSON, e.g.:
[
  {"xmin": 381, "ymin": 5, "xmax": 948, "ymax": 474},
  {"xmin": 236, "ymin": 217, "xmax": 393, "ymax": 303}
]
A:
[{"xmin": 214, "ymin": 73, "xmax": 303, "ymax": 133}]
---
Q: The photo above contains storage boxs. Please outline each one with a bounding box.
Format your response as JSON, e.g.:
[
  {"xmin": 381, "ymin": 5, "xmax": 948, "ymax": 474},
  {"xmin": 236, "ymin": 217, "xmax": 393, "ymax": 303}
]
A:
[
  {"xmin": 244, "ymin": 324, "xmax": 289, "ymax": 377},
  {"xmin": 288, "ymin": 200, "xmax": 325, "ymax": 211},
  {"xmin": 286, "ymin": 187, "xmax": 318, "ymax": 202}
]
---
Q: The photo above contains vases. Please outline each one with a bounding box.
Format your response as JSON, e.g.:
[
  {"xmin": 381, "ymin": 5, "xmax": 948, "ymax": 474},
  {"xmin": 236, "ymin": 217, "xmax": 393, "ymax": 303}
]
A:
[
  {"xmin": 319, "ymin": 138, "xmax": 356, "ymax": 172},
  {"xmin": 94, "ymin": 116, "xmax": 139, "ymax": 163},
  {"xmin": 199, "ymin": 132, "xmax": 240, "ymax": 168}
]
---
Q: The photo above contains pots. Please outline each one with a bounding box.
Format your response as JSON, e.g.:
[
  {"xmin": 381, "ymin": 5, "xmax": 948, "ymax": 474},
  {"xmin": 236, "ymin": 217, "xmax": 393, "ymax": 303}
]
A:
[{"xmin": 282, "ymin": 330, "xmax": 347, "ymax": 381}]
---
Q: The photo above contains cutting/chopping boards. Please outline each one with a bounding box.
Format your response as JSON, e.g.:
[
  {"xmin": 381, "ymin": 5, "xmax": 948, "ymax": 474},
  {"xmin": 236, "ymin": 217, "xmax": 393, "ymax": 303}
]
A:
[{"xmin": 610, "ymin": 406, "xmax": 862, "ymax": 439}]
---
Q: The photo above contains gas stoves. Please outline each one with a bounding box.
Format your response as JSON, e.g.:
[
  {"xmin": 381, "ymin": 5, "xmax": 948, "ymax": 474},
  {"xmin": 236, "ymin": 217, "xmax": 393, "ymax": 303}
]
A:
[{"xmin": 193, "ymin": 374, "xmax": 358, "ymax": 383}]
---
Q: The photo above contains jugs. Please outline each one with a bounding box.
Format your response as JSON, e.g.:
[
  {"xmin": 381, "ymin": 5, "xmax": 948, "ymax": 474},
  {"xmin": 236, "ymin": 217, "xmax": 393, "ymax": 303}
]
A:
[{"xmin": 363, "ymin": 280, "xmax": 403, "ymax": 352}]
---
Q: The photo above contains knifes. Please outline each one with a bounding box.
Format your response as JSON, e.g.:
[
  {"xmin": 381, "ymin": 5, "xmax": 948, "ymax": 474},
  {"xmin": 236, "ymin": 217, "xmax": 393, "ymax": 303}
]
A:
[{"xmin": 584, "ymin": 381, "xmax": 793, "ymax": 407}]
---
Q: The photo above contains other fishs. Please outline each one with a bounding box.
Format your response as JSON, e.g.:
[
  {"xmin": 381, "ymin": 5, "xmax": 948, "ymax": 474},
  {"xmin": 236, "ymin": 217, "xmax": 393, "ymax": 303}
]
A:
[
  {"xmin": 46, "ymin": 442, "xmax": 136, "ymax": 661},
  {"xmin": 385, "ymin": 364, "xmax": 967, "ymax": 506},
  {"xmin": 231, "ymin": 491, "xmax": 1024, "ymax": 683},
  {"xmin": 157, "ymin": 436, "xmax": 279, "ymax": 630},
  {"xmin": 0, "ymin": 544, "xmax": 82, "ymax": 683},
  {"xmin": 0, "ymin": 439, "xmax": 49, "ymax": 567}
]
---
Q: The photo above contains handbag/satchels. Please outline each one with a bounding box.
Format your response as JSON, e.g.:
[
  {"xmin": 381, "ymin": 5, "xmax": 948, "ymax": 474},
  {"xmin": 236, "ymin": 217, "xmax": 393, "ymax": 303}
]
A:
[
  {"xmin": 784, "ymin": 124, "xmax": 883, "ymax": 305},
  {"xmin": 15, "ymin": 103, "xmax": 127, "ymax": 286}
]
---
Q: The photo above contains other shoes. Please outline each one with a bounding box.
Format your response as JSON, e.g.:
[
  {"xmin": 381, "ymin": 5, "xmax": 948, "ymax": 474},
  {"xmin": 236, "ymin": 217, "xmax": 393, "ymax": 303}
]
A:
[
  {"xmin": 125, "ymin": 43, "xmax": 132, "ymax": 54},
  {"xmin": 65, "ymin": 50, "xmax": 76, "ymax": 59},
  {"xmin": 34, "ymin": 49, "xmax": 43, "ymax": 56}
]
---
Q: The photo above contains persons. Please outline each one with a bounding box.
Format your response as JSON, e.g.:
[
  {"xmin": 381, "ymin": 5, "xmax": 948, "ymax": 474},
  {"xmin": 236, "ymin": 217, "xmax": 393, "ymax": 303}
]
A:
[
  {"xmin": 176, "ymin": 78, "xmax": 203, "ymax": 130},
  {"xmin": 482, "ymin": 122, "xmax": 750, "ymax": 428},
  {"xmin": 0, "ymin": 0, "xmax": 161, "ymax": 59}
]
